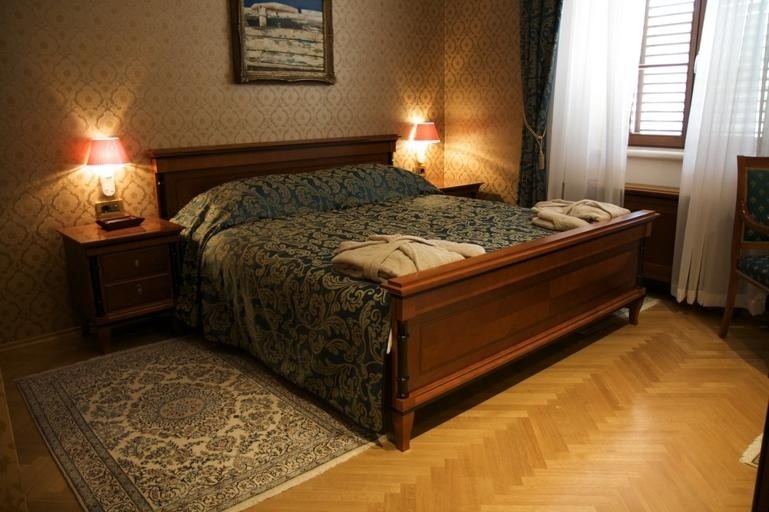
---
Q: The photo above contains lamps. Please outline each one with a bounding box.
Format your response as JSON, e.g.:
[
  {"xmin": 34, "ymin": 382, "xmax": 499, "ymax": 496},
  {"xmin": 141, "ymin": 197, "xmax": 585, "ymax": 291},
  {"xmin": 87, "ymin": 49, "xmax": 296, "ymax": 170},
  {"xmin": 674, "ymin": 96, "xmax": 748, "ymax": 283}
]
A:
[
  {"xmin": 414, "ymin": 122, "xmax": 441, "ymax": 165},
  {"xmin": 86, "ymin": 136, "xmax": 132, "ymax": 196}
]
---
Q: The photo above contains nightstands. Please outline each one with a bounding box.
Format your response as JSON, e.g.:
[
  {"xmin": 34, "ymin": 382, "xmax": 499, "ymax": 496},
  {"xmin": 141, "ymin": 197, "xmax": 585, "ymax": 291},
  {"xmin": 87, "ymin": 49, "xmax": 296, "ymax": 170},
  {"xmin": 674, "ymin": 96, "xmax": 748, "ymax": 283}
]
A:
[{"xmin": 54, "ymin": 216, "xmax": 187, "ymax": 345}]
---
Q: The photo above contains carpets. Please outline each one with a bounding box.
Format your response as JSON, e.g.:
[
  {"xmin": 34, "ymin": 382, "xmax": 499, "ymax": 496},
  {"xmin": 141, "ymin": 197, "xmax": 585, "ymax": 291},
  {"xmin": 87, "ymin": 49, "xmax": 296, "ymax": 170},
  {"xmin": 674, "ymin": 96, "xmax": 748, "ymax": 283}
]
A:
[{"xmin": 11, "ymin": 335, "xmax": 394, "ymax": 512}]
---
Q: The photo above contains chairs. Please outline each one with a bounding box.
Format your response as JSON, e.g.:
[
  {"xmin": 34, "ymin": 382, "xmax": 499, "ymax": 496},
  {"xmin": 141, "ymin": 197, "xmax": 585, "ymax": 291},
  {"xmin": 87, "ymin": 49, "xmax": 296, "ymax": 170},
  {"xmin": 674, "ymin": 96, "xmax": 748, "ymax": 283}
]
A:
[{"xmin": 717, "ymin": 154, "xmax": 769, "ymax": 340}]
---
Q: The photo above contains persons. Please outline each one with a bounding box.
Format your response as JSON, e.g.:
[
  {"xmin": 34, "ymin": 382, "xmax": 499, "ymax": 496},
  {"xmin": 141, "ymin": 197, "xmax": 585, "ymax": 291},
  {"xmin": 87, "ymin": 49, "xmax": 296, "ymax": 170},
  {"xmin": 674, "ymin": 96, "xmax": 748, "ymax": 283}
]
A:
[{"xmin": 256, "ymin": 5, "xmax": 268, "ymax": 31}]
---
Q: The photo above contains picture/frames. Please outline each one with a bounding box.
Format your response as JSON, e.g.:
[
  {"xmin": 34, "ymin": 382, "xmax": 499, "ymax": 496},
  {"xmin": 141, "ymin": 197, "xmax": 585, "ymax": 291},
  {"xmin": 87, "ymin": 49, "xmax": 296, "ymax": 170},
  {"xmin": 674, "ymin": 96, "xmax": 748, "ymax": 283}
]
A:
[{"xmin": 227, "ymin": 0, "xmax": 337, "ymax": 86}]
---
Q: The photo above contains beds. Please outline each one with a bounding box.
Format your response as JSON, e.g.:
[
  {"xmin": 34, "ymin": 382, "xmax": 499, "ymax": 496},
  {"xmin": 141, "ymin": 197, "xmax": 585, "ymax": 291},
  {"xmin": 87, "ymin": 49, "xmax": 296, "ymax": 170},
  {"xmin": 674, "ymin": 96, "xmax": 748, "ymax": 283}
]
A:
[{"xmin": 144, "ymin": 136, "xmax": 662, "ymax": 451}]
